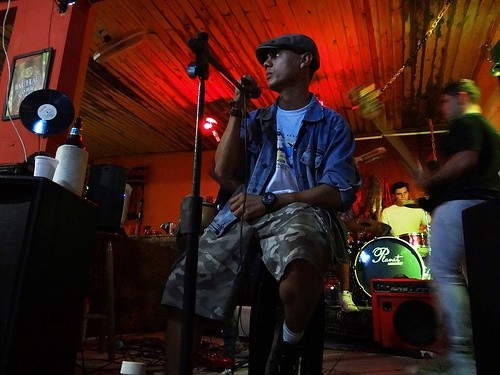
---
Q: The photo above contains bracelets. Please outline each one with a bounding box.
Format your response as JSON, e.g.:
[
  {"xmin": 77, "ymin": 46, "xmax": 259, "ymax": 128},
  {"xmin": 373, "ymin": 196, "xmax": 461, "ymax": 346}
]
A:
[{"xmin": 228, "ymin": 98, "xmax": 244, "ymax": 118}]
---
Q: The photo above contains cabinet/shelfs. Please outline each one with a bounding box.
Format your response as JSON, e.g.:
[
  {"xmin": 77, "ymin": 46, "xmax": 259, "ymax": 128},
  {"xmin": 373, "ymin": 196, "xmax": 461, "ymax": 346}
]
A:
[{"xmin": 0, "ymin": 174, "xmax": 98, "ymax": 375}]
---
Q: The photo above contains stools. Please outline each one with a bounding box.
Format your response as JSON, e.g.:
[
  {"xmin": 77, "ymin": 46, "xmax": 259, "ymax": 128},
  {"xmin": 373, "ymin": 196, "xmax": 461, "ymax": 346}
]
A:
[
  {"xmin": 234, "ymin": 240, "xmax": 325, "ymax": 374},
  {"xmin": 82, "ymin": 230, "xmax": 119, "ymax": 363}
]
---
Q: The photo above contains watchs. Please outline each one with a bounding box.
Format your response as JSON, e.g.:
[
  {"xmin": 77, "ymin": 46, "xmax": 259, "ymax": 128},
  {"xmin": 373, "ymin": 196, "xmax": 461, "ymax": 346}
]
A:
[{"xmin": 261, "ymin": 192, "xmax": 277, "ymax": 213}]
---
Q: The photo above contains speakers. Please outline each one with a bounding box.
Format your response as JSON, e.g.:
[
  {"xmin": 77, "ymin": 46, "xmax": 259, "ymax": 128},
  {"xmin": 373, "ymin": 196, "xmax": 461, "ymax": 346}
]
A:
[
  {"xmin": 373, "ymin": 293, "xmax": 446, "ymax": 355},
  {"xmin": 84, "ymin": 164, "xmax": 129, "ymax": 232}
]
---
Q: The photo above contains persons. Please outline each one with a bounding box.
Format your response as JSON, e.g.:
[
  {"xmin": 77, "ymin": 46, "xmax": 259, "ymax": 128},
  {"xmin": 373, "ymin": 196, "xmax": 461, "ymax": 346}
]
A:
[
  {"xmin": 381, "ymin": 182, "xmax": 432, "ymax": 238},
  {"xmin": 423, "ymin": 78, "xmax": 500, "ymax": 375},
  {"xmin": 160, "ymin": 35, "xmax": 380, "ymax": 375}
]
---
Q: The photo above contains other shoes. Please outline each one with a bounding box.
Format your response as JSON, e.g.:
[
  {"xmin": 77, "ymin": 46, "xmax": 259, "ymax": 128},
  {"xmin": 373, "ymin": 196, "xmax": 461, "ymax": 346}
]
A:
[{"xmin": 265, "ymin": 315, "xmax": 303, "ymax": 375}]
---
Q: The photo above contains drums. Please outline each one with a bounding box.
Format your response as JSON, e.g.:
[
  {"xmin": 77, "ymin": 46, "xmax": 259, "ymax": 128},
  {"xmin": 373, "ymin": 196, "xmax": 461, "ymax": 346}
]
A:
[
  {"xmin": 398, "ymin": 232, "xmax": 429, "ymax": 256},
  {"xmin": 351, "ymin": 235, "xmax": 426, "ymax": 299}
]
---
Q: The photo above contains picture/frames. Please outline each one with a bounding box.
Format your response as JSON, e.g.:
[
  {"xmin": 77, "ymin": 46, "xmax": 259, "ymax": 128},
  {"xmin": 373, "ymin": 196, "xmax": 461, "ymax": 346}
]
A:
[{"xmin": 2, "ymin": 46, "xmax": 54, "ymax": 121}]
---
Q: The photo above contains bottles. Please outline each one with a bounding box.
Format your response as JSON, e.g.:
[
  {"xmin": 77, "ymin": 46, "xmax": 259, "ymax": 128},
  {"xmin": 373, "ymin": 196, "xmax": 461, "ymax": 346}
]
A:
[{"xmin": 63, "ymin": 116, "xmax": 86, "ymax": 149}]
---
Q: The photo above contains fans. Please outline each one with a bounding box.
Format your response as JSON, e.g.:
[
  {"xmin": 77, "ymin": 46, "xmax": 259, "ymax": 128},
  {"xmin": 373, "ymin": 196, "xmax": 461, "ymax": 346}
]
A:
[{"xmin": 92, "ymin": 29, "xmax": 146, "ymax": 63}]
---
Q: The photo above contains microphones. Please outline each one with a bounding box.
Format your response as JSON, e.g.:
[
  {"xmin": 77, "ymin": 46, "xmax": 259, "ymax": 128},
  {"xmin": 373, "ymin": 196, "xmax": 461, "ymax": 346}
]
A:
[{"xmin": 240, "ymin": 78, "xmax": 262, "ymax": 98}]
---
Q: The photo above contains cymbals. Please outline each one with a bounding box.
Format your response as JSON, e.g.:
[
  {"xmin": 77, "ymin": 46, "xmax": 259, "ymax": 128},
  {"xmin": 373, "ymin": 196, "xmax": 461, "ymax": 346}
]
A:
[
  {"xmin": 402, "ymin": 202, "xmax": 421, "ymax": 208},
  {"xmin": 349, "ymin": 222, "xmax": 392, "ymax": 233}
]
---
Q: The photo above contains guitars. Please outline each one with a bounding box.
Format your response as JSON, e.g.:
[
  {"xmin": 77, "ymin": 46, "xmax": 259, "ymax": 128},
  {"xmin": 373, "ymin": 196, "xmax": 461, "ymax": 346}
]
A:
[
  {"xmin": 354, "ymin": 83, "xmax": 446, "ymax": 215},
  {"xmin": 353, "ymin": 146, "xmax": 386, "ymax": 166}
]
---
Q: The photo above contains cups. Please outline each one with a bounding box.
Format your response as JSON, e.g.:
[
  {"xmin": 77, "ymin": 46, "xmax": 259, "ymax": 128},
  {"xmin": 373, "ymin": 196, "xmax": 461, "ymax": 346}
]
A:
[
  {"xmin": 120, "ymin": 361, "xmax": 147, "ymax": 375},
  {"xmin": 33, "ymin": 156, "xmax": 59, "ymax": 180},
  {"xmin": 52, "ymin": 145, "xmax": 89, "ymax": 197}
]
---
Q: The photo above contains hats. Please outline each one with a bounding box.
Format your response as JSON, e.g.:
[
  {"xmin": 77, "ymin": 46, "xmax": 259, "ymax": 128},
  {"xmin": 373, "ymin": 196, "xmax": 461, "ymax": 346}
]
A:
[{"xmin": 255, "ymin": 33, "xmax": 320, "ymax": 71}]
